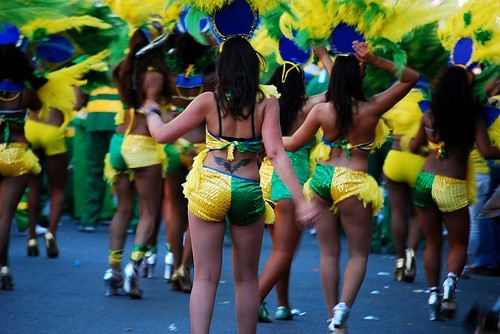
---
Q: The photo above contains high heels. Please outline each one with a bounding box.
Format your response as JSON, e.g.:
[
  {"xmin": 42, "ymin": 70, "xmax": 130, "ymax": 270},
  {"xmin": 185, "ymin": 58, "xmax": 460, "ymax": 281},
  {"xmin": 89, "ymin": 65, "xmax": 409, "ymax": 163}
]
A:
[
  {"xmin": 171, "ymin": 266, "xmax": 194, "ymax": 294},
  {"xmin": 0, "ymin": 266, "xmax": 13, "ymax": 292},
  {"xmin": 428, "ymin": 273, "xmax": 458, "ymax": 323},
  {"xmin": 103, "ymin": 263, "xmax": 144, "ymax": 301},
  {"xmin": 395, "ymin": 248, "xmax": 416, "ymax": 284},
  {"xmin": 140, "ymin": 253, "xmax": 182, "ymax": 281},
  {"xmin": 27, "ymin": 232, "xmax": 60, "ymax": 260},
  {"xmin": 327, "ymin": 302, "xmax": 350, "ymax": 334}
]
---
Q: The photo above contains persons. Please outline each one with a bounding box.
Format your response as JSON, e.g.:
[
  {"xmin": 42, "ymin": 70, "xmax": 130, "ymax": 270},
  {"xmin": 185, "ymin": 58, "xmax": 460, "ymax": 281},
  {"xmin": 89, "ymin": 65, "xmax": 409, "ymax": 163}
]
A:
[
  {"xmin": 14, "ymin": 0, "xmax": 500, "ymax": 278},
  {"xmin": 408, "ymin": 0, "xmax": 500, "ymax": 323},
  {"xmin": 100, "ymin": 0, "xmax": 194, "ymax": 300},
  {"xmin": 0, "ymin": 0, "xmax": 112, "ymax": 290},
  {"xmin": 265, "ymin": 0, "xmax": 460, "ymax": 334},
  {"xmin": 137, "ymin": 1, "xmax": 322, "ymax": 334}
]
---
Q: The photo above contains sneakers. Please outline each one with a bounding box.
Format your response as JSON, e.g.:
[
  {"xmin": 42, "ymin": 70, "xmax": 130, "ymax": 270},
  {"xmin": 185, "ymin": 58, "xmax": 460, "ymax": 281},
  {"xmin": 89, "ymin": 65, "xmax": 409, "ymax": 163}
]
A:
[
  {"xmin": 125, "ymin": 228, "xmax": 135, "ymax": 234},
  {"xmin": 257, "ymin": 302, "xmax": 272, "ymax": 323},
  {"xmin": 275, "ymin": 306, "xmax": 293, "ymax": 321},
  {"xmin": 25, "ymin": 223, "xmax": 49, "ymax": 235},
  {"xmin": 76, "ymin": 224, "xmax": 95, "ymax": 233},
  {"xmin": 100, "ymin": 219, "xmax": 115, "ymax": 226}
]
